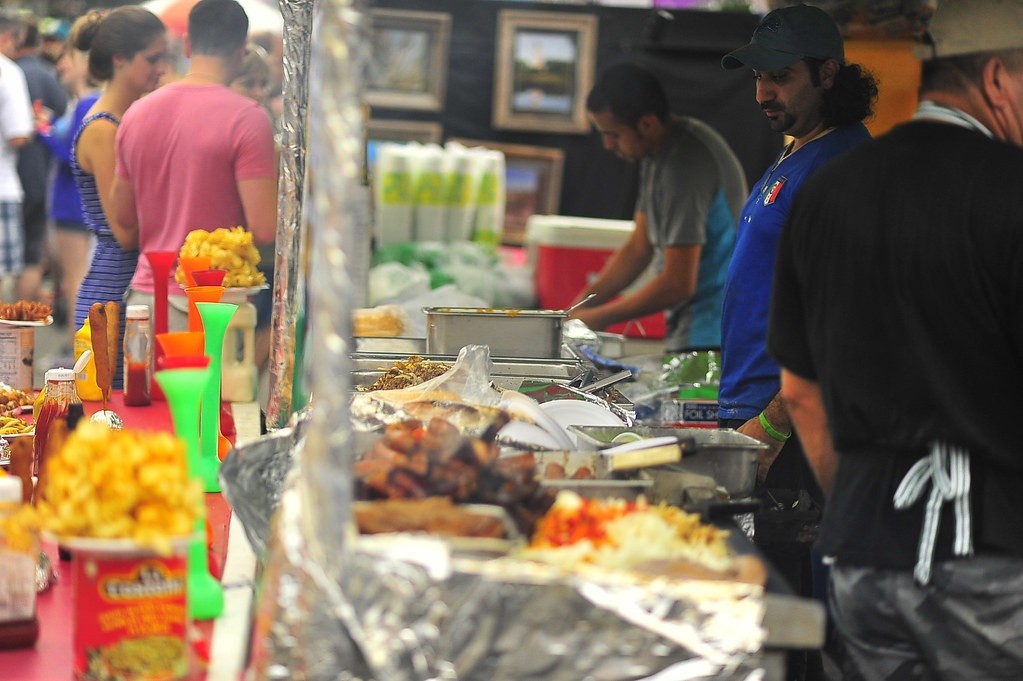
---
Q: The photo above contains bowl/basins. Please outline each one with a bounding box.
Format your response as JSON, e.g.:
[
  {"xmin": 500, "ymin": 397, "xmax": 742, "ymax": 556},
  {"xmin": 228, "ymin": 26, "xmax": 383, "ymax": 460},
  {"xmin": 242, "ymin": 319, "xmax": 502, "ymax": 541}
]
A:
[
  {"xmin": 420, "ymin": 304, "xmax": 570, "ymax": 358},
  {"xmin": 509, "ymin": 427, "xmax": 771, "ymax": 516}
]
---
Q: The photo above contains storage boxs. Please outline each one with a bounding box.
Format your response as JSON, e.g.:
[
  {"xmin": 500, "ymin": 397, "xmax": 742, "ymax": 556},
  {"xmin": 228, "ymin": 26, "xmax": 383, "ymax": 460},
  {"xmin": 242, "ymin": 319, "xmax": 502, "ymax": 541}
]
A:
[
  {"xmin": 526, "ymin": 215, "xmax": 665, "ymax": 339},
  {"xmin": 566, "ymin": 412, "xmax": 770, "ymax": 500},
  {"xmin": 420, "ymin": 302, "xmax": 568, "ymax": 359}
]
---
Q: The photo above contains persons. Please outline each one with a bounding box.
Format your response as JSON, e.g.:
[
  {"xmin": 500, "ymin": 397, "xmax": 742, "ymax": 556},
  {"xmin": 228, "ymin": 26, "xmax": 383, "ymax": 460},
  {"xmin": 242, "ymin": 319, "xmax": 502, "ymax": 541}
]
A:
[
  {"xmin": 568, "ymin": 60, "xmax": 749, "ymax": 356},
  {"xmin": 762, "ymin": 0, "xmax": 1023, "ymax": 681},
  {"xmin": 711, "ymin": 4, "xmax": 884, "ymax": 598},
  {"xmin": 0, "ymin": 0, "xmax": 287, "ymax": 389}
]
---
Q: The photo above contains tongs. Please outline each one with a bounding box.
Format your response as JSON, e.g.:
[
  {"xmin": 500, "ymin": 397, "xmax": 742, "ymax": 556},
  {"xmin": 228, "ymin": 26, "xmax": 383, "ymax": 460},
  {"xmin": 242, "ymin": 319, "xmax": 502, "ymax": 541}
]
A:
[{"xmin": 567, "ymin": 368, "xmax": 632, "ymax": 393}]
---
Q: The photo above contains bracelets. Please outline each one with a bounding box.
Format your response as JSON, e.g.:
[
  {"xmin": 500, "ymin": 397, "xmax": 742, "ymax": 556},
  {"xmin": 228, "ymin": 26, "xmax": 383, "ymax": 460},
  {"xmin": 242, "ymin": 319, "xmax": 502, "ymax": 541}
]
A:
[{"xmin": 758, "ymin": 411, "xmax": 792, "ymax": 441}]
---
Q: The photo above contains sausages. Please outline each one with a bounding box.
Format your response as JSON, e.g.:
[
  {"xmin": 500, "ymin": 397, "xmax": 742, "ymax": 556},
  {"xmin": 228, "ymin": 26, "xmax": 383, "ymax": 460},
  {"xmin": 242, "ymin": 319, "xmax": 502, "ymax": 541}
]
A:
[{"xmin": 90, "ymin": 301, "xmax": 120, "ymax": 389}]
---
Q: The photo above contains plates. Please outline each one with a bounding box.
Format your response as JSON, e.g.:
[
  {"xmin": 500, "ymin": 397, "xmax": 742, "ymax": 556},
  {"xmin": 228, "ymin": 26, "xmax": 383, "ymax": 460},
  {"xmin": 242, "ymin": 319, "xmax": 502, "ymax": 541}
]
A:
[
  {"xmin": 223, "ymin": 284, "xmax": 272, "ymax": 291},
  {"xmin": 0, "ymin": 317, "xmax": 56, "ymax": 328},
  {"xmin": 35, "ymin": 530, "xmax": 206, "ymax": 557},
  {"xmin": 499, "ymin": 397, "xmax": 628, "ymax": 451}
]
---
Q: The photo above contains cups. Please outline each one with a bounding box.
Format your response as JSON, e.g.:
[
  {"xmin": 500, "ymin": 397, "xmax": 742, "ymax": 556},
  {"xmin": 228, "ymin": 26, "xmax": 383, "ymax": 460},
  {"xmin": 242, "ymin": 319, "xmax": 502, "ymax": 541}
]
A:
[{"xmin": 374, "ymin": 140, "xmax": 507, "ymax": 246}]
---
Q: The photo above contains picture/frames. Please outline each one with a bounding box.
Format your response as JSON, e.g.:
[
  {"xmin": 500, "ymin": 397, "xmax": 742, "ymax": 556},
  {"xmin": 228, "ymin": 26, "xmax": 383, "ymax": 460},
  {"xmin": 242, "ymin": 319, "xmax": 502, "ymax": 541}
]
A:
[
  {"xmin": 451, "ymin": 137, "xmax": 567, "ymax": 251},
  {"xmin": 362, "ymin": 9, "xmax": 453, "ymax": 111},
  {"xmin": 366, "ymin": 121, "xmax": 441, "ymax": 237},
  {"xmin": 491, "ymin": 9, "xmax": 599, "ymax": 136}
]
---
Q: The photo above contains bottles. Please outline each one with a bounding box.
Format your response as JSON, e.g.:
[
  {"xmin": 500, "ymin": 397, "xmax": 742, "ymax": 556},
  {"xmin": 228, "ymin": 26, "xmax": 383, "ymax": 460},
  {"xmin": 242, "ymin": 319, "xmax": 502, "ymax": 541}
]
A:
[
  {"xmin": 122, "ymin": 305, "xmax": 154, "ymax": 407},
  {"xmin": 0, "ymin": 474, "xmax": 41, "ymax": 647},
  {"xmin": 34, "ymin": 351, "xmax": 93, "ymax": 485},
  {"xmin": 72, "ymin": 316, "xmax": 112, "ymax": 401}
]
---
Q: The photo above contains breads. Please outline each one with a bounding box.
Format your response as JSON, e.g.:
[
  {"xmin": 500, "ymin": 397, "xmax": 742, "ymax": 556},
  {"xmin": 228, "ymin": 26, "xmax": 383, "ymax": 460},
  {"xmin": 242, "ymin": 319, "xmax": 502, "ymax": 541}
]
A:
[{"xmin": 354, "ymin": 308, "xmax": 404, "ymax": 337}]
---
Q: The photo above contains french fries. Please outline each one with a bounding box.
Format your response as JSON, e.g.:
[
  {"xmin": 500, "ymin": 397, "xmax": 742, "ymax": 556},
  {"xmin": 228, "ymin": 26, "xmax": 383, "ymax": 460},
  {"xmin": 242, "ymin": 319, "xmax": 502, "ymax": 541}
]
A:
[{"xmin": 0, "ymin": 415, "xmax": 36, "ymax": 434}]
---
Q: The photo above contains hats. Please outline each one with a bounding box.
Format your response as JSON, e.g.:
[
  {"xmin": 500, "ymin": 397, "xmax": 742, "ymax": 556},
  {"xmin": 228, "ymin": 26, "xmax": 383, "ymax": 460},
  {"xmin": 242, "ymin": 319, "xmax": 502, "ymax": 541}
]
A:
[
  {"xmin": 915, "ymin": 0, "xmax": 1023, "ymax": 60},
  {"xmin": 720, "ymin": 4, "xmax": 844, "ymax": 72}
]
